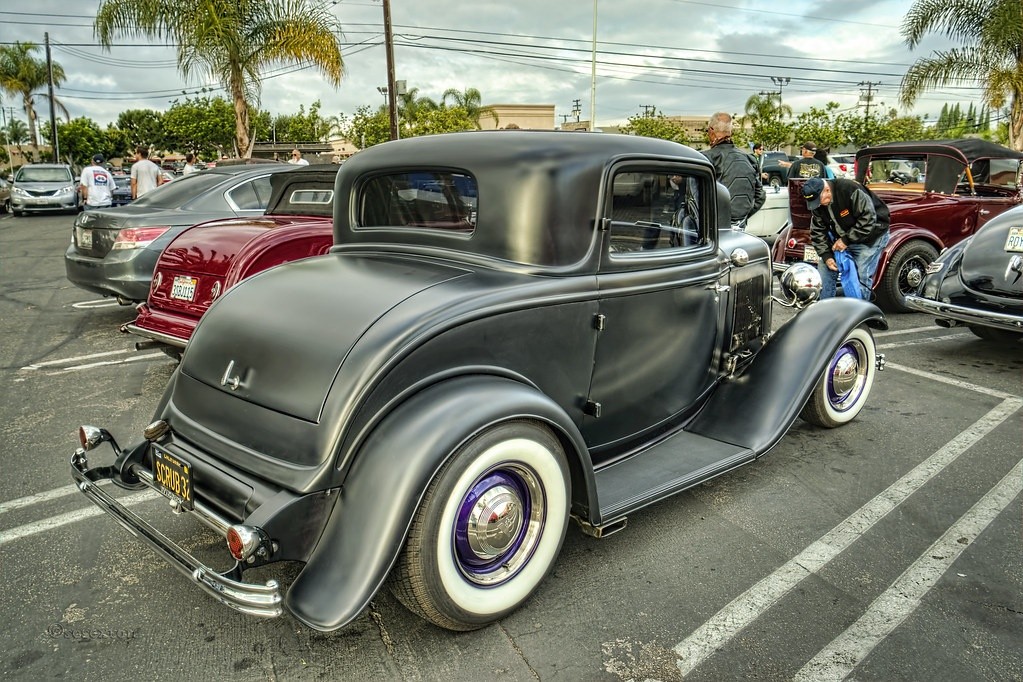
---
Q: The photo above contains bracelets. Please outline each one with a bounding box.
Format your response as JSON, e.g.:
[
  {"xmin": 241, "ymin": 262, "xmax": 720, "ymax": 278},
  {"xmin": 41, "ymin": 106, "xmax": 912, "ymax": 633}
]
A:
[{"xmin": 83, "ymin": 198, "xmax": 87, "ymax": 200}]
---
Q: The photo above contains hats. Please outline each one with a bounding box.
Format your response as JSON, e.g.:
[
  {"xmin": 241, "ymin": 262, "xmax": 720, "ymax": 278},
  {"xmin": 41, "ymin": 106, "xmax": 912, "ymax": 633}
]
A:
[
  {"xmin": 801, "ymin": 177, "xmax": 824, "ymax": 210},
  {"xmin": 92, "ymin": 154, "xmax": 105, "ymax": 164},
  {"xmin": 799, "ymin": 141, "xmax": 818, "ymax": 152}
]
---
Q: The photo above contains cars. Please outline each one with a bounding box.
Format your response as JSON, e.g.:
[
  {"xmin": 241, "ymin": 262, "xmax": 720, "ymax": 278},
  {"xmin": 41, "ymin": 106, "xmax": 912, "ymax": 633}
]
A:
[
  {"xmin": 699, "ymin": 137, "xmax": 1023, "ymax": 344},
  {"xmin": 0, "ymin": 159, "xmax": 473, "ymax": 362},
  {"xmin": 69, "ymin": 129, "xmax": 890, "ymax": 635}
]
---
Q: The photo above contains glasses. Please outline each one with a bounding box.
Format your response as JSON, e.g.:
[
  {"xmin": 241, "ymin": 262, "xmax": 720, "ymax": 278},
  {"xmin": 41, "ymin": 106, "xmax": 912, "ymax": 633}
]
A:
[
  {"xmin": 705, "ymin": 127, "xmax": 714, "ymax": 135},
  {"xmin": 757, "ymin": 148, "xmax": 765, "ymax": 151},
  {"xmin": 134, "ymin": 152, "xmax": 141, "ymax": 155},
  {"xmin": 291, "ymin": 154, "xmax": 297, "ymax": 157}
]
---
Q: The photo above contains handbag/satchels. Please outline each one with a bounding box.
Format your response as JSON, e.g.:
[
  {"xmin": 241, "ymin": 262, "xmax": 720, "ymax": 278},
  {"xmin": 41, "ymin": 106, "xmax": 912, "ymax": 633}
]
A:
[{"xmin": 833, "ymin": 250, "xmax": 876, "ymax": 303}]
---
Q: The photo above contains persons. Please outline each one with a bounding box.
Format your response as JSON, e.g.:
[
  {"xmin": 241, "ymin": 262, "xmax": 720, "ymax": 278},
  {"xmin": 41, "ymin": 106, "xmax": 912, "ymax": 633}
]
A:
[
  {"xmin": 673, "ymin": 113, "xmax": 765, "ymax": 230},
  {"xmin": 777, "ymin": 142, "xmax": 836, "ymax": 178},
  {"xmin": 749, "ymin": 143, "xmax": 767, "ymax": 184},
  {"xmin": 131, "ymin": 147, "xmax": 162, "ymax": 200},
  {"xmin": 80, "ymin": 154, "xmax": 116, "ymax": 209},
  {"xmin": 804, "ymin": 179, "xmax": 889, "ymax": 300},
  {"xmin": 183, "ymin": 154, "xmax": 201, "ymax": 175},
  {"xmin": 288, "ymin": 150, "xmax": 309, "ymax": 165}
]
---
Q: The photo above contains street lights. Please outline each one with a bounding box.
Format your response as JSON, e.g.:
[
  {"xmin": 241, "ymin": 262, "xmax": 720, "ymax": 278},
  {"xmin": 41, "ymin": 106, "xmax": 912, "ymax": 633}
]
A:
[
  {"xmin": 770, "ymin": 77, "xmax": 790, "ymax": 150},
  {"xmin": 377, "ymin": 86, "xmax": 388, "ymax": 103}
]
